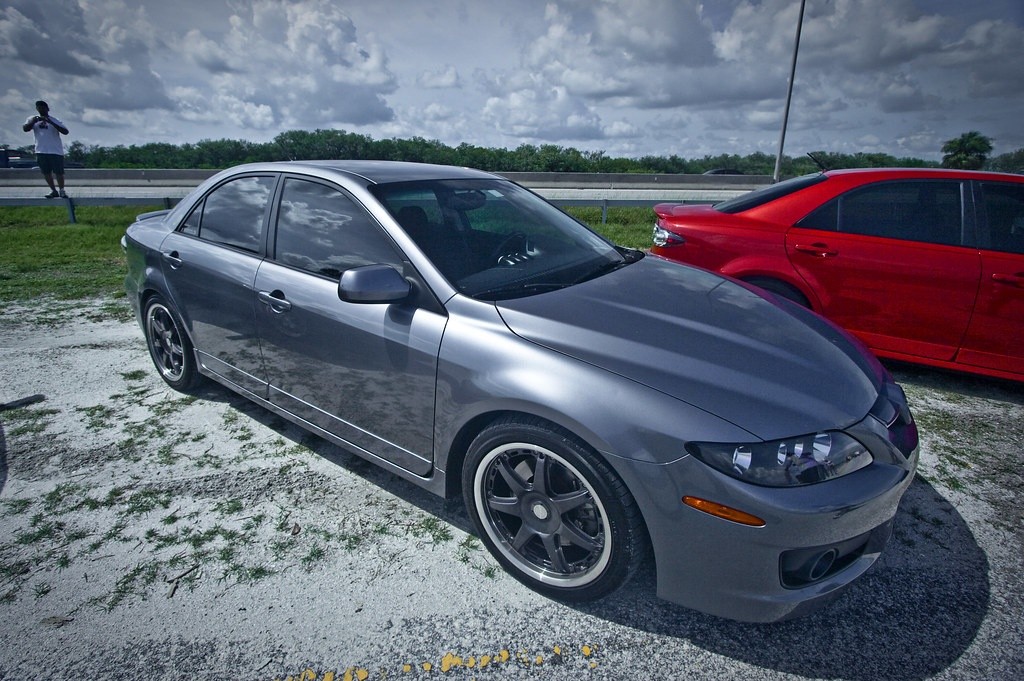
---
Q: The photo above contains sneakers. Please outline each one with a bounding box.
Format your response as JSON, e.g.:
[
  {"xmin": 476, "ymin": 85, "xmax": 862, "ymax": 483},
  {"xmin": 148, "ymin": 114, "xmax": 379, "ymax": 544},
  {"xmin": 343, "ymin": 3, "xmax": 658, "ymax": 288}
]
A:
[
  {"xmin": 60, "ymin": 191, "xmax": 68, "ymax": 198},
  {"xmin": 45, "ymin": 191, "xmax": 59, "ymax": 198}
]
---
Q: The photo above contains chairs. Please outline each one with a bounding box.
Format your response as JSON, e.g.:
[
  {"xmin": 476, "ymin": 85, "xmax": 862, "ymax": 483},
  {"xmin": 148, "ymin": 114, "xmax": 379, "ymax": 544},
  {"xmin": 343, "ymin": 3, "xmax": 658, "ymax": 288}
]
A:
[
  {"xmin": 989, "ymin": 201, "xmax": 1024, "ymax": 252},
  {"xmin": 938, "ymin": 195, "xmax": 961, "ymax": 245},
  {"xmin": 397, "ymin": 206, "xmax": 461, "ymax": 274}
]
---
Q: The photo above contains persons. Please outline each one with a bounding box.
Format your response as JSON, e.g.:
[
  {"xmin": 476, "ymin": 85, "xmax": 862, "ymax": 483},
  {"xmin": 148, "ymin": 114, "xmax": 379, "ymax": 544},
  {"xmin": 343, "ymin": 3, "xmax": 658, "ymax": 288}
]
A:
[{"xmin": 23, "ymin": 101, "xmax": 70, "ymax": 198}]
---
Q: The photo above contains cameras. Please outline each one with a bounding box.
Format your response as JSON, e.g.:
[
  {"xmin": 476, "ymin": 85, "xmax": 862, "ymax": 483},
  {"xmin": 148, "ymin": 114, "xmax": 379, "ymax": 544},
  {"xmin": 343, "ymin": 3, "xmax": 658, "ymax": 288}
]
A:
[{"xmin": 36, "ymin": 116, "xmax": 44, "ymax": 121}]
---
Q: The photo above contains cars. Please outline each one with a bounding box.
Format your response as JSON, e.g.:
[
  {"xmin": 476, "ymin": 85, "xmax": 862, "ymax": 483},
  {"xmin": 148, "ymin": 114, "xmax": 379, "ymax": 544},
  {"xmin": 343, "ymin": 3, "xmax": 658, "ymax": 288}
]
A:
[
  {"xmin": 118, "ymin": 157, "xmax": 922, "ymax": 626},
  {"xmin": 703, "ymin": 168, "xmax": 744, "ymax": 175},
  {"xmin": 649, "ymin": 165, "xmax": 1024, "ymax": 384}
]
